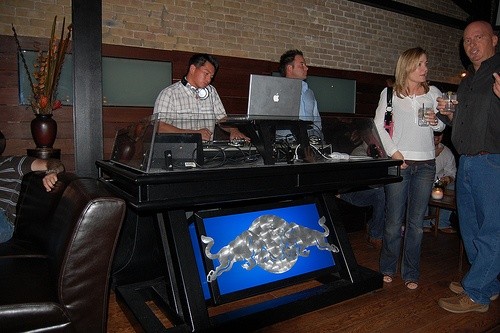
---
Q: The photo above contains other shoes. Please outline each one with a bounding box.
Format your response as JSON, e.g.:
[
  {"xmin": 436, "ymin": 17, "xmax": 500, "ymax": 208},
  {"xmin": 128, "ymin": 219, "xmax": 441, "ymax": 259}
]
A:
[
  {"xmin": 431, "ymin": 221, "xmax": 457, "ymax": 234},
  {"xmin": 423, "ymin": 227, "xmax": 432, "ymax": 233},
  {"xmin": 367, "ymin": 236, "xmax": 383, "ymax": 250}
]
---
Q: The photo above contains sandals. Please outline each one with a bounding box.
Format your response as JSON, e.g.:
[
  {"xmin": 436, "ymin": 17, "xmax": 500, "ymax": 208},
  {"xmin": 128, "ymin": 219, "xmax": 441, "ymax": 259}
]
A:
[
  {"xmin": 405, "ymin": 280, "xmax": 418, "ymax": 289},
  {"xmin": 381, "ymin": 272, "xmax": 393, "ymax": 284}
]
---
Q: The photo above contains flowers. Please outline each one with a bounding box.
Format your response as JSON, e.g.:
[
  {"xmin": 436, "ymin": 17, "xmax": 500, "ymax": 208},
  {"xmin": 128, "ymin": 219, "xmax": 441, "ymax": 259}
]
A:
[{"xmin": 10, "ymin": 15, "xmax": 72, "ymax": 113}]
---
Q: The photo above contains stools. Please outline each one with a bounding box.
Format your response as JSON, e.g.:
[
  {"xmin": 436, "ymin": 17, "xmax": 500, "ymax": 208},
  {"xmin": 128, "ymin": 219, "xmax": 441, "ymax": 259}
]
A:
[{"xmin": 332, "ymin": 198, "xmax": 376, "ymax": 228}]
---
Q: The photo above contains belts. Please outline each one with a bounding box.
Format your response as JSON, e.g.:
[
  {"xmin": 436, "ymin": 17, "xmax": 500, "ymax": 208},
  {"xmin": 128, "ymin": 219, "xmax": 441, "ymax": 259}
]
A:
[{"xmin": 388, "ymin": 156, "xmax": 435, "ymax": 165}]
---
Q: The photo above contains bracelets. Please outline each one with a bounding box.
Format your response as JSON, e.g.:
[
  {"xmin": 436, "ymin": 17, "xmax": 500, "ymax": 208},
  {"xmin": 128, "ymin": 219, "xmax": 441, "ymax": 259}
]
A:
[{"xmin": 46, "ymin": 169, "xmax": 56, "ymax": 174}]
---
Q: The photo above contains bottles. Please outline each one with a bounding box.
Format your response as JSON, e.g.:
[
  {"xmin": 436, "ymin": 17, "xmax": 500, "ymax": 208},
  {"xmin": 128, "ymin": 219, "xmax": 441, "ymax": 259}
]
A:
[{"xmin": 432, "ymin": 187, "xmax": 443, "ymax": 199}]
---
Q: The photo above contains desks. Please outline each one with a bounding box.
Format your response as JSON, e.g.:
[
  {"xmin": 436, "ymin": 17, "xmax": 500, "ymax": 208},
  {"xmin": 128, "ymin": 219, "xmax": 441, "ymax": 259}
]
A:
[{"xmin": 423, "ymin": 191, "xmax": 468, "ymax": 274}]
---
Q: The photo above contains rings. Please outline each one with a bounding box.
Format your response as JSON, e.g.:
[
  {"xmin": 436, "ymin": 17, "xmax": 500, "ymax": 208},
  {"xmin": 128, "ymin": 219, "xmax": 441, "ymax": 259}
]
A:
[{"xmin": 435, "ymin": 119, "xmax": 438, "ymax": 122}]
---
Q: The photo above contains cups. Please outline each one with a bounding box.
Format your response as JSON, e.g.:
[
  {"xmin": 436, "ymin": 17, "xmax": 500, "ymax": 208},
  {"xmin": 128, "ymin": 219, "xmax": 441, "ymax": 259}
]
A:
[
  {"xmin": 442, "ymin": 92, "xmax": 456, "ymax": 113},
  {"xmin": 423, "ymin": 102, "xmax": 433, "ymax": 125}
]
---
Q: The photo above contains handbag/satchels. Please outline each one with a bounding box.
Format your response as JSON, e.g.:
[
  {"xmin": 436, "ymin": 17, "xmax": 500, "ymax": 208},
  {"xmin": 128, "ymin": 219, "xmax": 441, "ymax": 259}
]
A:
[{"xmin": 367, "ymin": 85, "xmax": 394, "ymax": 158}]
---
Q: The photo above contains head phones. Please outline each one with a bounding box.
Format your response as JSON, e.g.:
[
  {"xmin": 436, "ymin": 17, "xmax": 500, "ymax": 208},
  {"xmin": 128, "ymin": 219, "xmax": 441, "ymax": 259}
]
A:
[{"xmin": 181, "ymin": 77, "xmax": 208, "ymax": 100}]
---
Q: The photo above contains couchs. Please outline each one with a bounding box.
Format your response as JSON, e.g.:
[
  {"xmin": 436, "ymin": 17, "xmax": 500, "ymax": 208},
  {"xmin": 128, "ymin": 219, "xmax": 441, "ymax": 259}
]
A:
[{"xmin": 0, "ymin": 171, "xmax": 126, "ymax": 333}]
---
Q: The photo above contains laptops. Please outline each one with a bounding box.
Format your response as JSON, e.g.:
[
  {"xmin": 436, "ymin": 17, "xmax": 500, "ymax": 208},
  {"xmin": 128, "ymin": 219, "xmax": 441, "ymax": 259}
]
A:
[{"xmin": 226, "ymin": 74, "xmax": 302, "ymax": 120}]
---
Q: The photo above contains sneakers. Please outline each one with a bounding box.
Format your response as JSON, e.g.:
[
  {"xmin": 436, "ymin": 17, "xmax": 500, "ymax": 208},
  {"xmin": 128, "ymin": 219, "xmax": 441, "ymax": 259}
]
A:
[
  {"xmin": 449, "ymin": 281, "xmax": 499, "ymax": 304},
  {"xmin": 438, "ymin": 291, "xmax": 490, "ymax": 314}
]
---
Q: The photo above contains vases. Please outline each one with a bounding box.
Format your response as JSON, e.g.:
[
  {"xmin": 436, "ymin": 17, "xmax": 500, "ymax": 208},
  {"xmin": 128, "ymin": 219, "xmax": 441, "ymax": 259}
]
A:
[{"xmin": 30, "ymin": 114, "xmax": 58, "ymax": 148}]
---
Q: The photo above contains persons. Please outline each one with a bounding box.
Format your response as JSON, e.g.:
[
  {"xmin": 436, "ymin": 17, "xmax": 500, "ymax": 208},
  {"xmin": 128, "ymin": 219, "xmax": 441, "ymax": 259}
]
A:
[
  {"xmin": 336, "ymin": 134, "xmax": 387, "ymax": 245},
  {"xmin": 438, "ymin": 21, "xmax": 500, "ymax": 313},
  {"xmin": 0, "ymin": 131, "xmax": 65, "ymax": 243},
  {"xmin": 153, "ymin": 53, "xmax": 250, "ymax": 142},
  {"xmin": 373, "ymin": 47, "xmax": 447, "ymax": 290},
  {"xmin": 433, "ymin": 131, "xmax": 456, "ymax": 187},
  {"xmin": 280, "ymin": 48, "xmax": 327, "ymax": 154}
]
